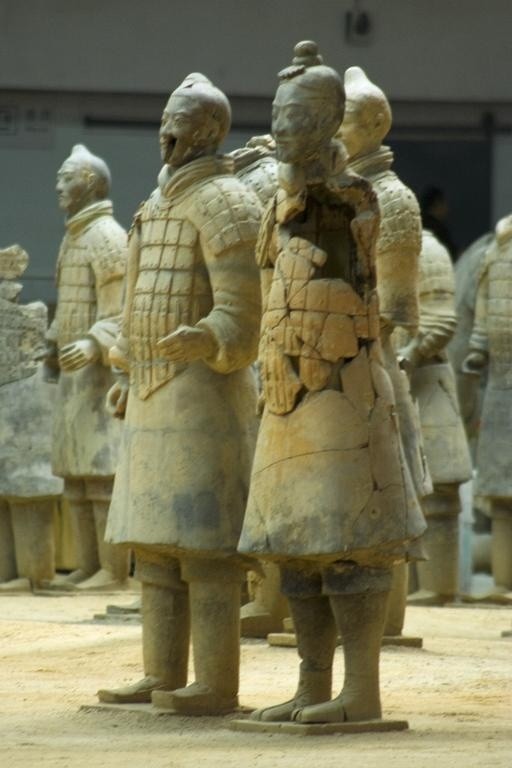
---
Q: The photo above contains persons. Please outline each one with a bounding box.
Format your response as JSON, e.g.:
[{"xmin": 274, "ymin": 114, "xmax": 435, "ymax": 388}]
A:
[{"xmin": 1, "ymin": 40, "xmax": 512, "ymax": 730}]
